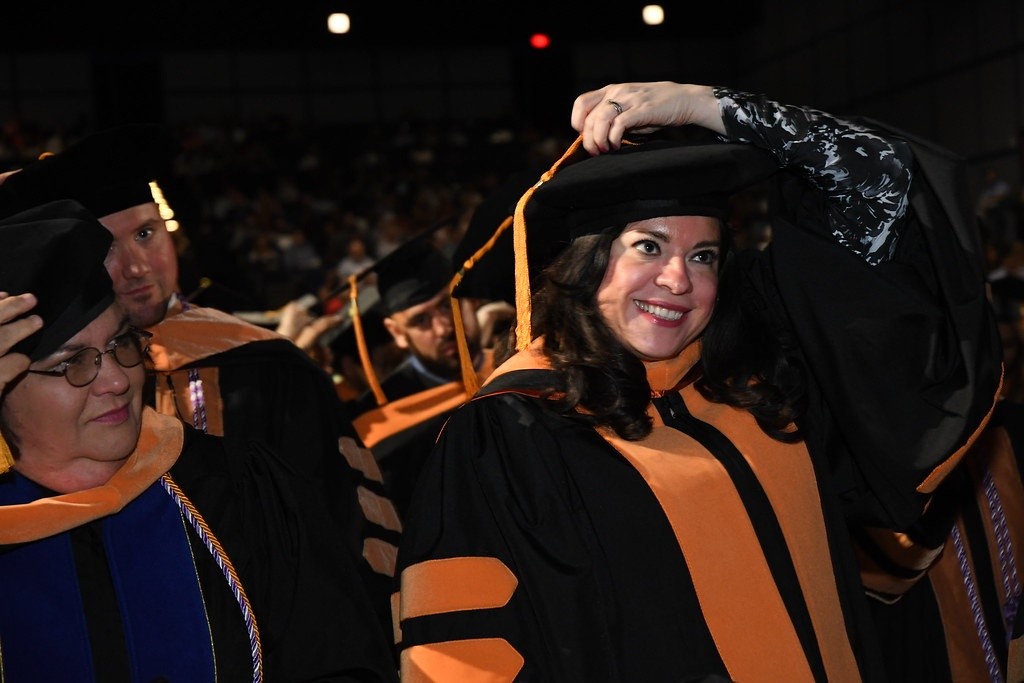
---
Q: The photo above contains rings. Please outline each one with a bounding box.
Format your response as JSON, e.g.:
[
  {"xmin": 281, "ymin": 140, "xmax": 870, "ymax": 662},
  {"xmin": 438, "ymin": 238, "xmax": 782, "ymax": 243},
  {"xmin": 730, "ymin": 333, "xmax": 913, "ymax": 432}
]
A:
[{"xmin": 609, "ymin": 100, "xmax": 622, "ymax": 114}]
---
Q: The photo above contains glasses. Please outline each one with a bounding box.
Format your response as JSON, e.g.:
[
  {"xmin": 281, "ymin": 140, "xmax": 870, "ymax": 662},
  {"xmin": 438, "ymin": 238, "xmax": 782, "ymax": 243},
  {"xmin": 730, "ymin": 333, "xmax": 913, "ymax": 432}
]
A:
[{"xmin": 26, "ymin": 323, "xmax": 154, "ymax": 388}]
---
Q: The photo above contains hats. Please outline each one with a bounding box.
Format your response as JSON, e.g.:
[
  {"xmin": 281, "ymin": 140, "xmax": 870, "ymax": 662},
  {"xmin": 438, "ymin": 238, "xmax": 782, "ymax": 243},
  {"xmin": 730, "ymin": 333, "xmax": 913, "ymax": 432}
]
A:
[
  {"xmin": 530, "ymin": 124, "xmax": 757, "ymax": 233},
  {"xmin": 314, "ymin": 217, "xmax": 450, "ymax": 408},
  {"xmin": 448, "ymin": 192, "xmax": 553, "ymax": 399},
  {"xmin": 0, "ymin": 149, "xmax": 155, "ymax": 362}
]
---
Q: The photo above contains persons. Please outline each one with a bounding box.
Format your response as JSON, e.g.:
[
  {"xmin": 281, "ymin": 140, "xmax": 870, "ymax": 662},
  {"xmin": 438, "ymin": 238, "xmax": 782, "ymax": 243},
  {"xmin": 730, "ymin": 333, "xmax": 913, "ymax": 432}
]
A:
[
  {"xmin": 730, "ymin": 196, "xmax": 1024, "ymax": 683},
  {"xmin": 0, "ymin": 200, "xmax": 397, "ymax": 683},
  {"xmin": 0, "ymin": 108, "xmax": 527, "ymax": 669},
  {"xmin": 387, "ymin": 82, "xmax": 1003, "ymax": 683}
]
